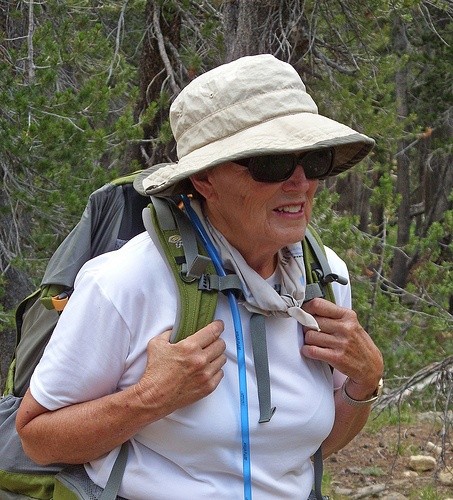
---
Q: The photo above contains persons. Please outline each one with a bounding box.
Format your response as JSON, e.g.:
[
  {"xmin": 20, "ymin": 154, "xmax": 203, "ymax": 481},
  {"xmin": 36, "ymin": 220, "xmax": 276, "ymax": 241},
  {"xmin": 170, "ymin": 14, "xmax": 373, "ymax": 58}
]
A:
[{"xmin": 15, "ymin": 52, "xmax": 384, "ymax": 500}]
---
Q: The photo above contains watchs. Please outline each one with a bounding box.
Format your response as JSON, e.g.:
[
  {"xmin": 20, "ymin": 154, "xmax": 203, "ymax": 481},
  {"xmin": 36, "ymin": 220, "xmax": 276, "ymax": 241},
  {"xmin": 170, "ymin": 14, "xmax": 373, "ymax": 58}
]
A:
[{"xmin": 342, "ymin": 377, "xmax": 384, "ymax": 408}]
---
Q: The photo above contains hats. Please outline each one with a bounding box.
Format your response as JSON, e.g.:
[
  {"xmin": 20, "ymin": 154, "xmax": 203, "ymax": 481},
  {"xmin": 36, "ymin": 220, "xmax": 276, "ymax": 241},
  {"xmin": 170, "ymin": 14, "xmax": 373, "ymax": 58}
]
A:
[{"xmin": 132, "ymin": 55, "xmax": 375, "ymax": 200}]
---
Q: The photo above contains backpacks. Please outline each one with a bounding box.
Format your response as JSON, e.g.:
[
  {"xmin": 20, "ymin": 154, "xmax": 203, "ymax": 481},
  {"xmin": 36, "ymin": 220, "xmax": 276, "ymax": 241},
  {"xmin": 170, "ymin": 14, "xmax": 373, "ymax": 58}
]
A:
[{"xmin": 0, "ymin": 163, "xmax": 176, "ymax": 500}]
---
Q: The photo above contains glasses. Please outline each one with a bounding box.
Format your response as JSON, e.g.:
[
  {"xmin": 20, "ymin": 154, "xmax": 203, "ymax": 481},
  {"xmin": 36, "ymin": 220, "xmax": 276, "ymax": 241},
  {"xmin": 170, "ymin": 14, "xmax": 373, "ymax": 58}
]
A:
[{"xmin": 235, "ymin": 146, "xmax": 336, "ymax": 185}]
